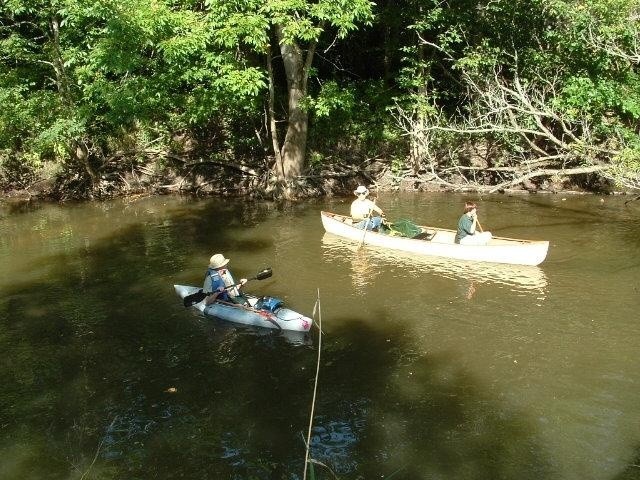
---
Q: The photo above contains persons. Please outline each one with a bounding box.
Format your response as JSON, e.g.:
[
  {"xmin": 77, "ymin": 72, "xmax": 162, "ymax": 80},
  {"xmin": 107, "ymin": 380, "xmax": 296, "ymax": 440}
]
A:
[
  {"xmin": 202, "ymin": 254, "xmax": 251, "ymax": 308},
  {"xmin": 351, "ymin": 186, "xmax": 386, "ymax": 233},
  {"xmin": 456, "ymin": 201, "xmax": 492, "ymax": 245}
]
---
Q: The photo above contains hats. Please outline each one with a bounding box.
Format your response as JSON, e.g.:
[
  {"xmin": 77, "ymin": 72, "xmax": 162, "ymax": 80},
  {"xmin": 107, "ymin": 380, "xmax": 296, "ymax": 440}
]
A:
[
  {"xmin": 208, "ymin": 253, "xmax": 231, "ymax": 270},
  {"xmin": 353, "ymin": 186, "xmax": 370, "ymax": 197}
]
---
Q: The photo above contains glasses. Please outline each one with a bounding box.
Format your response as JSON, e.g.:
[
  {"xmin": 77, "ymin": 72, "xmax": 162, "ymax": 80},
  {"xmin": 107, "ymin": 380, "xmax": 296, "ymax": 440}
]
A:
[{"xmin": 357, "ymin": 192, "xmax": 367, "ymax": 196}]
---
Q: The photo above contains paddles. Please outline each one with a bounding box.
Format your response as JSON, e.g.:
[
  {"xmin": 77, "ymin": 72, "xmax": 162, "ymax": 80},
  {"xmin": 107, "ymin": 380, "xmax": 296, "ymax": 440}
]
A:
[
  {"xmin": 183, "ymin": 267, "xmax": 272, "ymax": 307},
  {"xmin": 357, "ymin": 198, "xmax": 377, "ymax": 251}
]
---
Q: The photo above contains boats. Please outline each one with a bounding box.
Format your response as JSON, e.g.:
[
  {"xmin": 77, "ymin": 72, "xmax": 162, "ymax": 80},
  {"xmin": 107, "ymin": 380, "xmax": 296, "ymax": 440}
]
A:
[
  {"xmin": 318, "ymin": 232, "xmax": 550, "ymax": 297},
  {"xmin": 171, "ymin": 282, "xmax": 314, "ymax": 336},
  {"xmin": 317, "ymin": 209, "xmax": 555, "ymax": 269}
]
